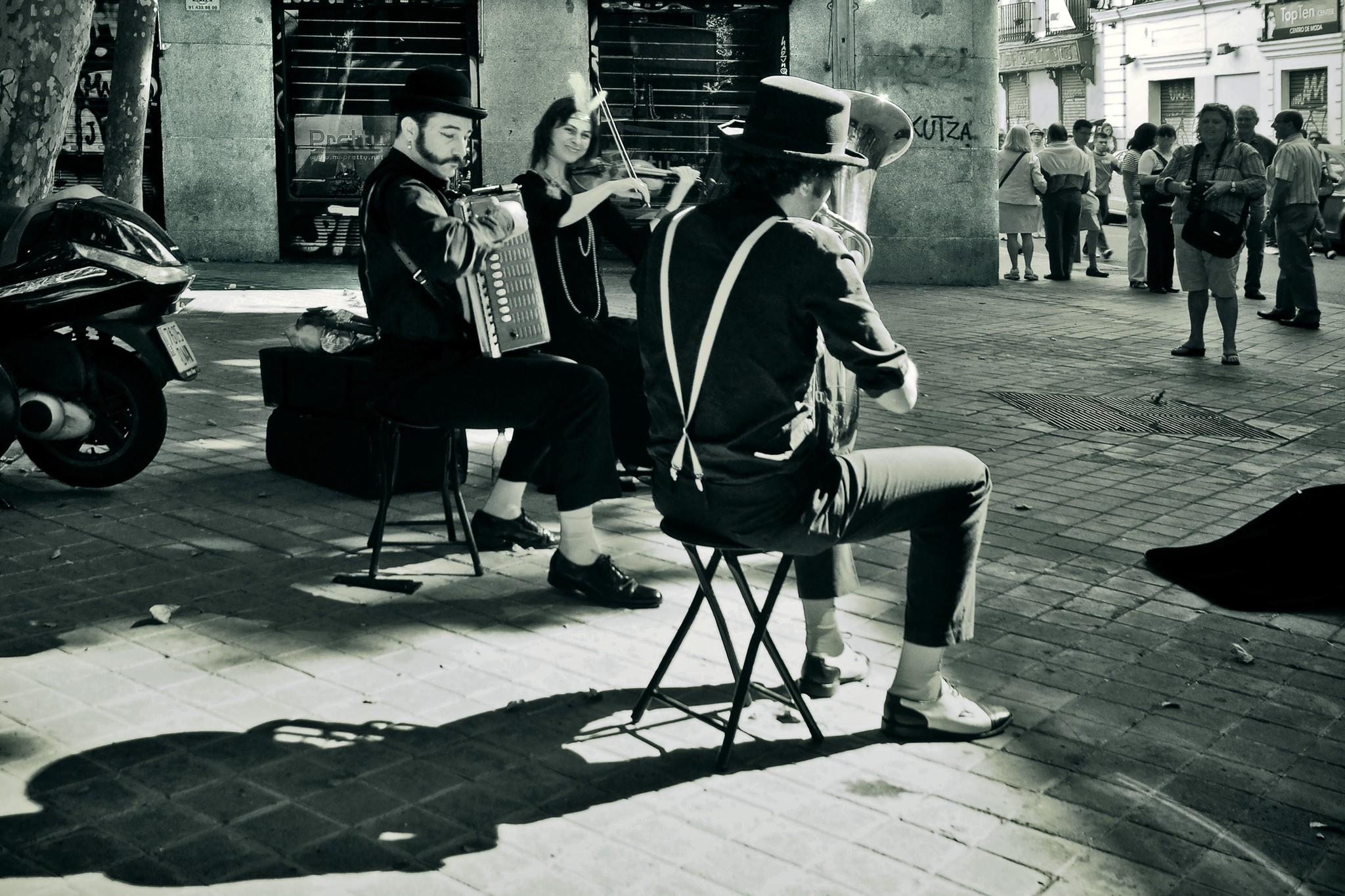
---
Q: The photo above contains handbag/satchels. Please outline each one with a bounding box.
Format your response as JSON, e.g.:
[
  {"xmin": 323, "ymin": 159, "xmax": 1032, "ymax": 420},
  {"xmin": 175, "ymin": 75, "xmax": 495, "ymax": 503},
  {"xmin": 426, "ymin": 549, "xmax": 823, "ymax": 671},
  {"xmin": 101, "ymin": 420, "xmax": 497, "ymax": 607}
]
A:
[{"xmin": 1181, "ymin": 208, "xmax": 1244, "ymax": 259}]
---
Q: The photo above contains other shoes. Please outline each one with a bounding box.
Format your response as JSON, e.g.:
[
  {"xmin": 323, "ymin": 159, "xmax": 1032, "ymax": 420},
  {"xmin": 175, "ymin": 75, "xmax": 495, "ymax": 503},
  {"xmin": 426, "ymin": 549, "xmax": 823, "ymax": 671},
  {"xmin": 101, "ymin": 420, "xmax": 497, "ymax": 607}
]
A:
[
  {"xmin": 1130, "ymin": 281, "xmax": 1148, "ymax": 289},
  {"xmin": 1327, "ymin": 250, "xmax": 1337, "ymax": 259},
  {"xmin": 1037, "ymin": 232, "xmax": 1046, "ymax": 238},
  {"xmin": 1103, "ymin": 249, "xmax": 1113, "ymax": 259},
  {"xmin": 1031, "ymin": 232, "xmax": 1039, "ymax": 238},
  {"xmin": 1309, "ymin": 247, "xmax": 1316, "ymax": 257},
  {"xmin": 1083, "ymin": 253, "xmax": 1100, "ymax": 257},
  {"xmin": 1264, "ymin": 247, "xmax": 1279, "ymax": 254},
  {"xmin": 1000, "ymin": 236, "xmax": 1008, "ymax": 241}
]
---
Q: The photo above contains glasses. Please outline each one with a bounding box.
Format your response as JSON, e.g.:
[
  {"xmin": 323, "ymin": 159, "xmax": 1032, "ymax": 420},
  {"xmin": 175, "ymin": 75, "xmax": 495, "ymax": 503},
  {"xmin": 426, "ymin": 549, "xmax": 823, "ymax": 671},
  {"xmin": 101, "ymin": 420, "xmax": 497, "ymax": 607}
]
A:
[
  {"xmin": 1274, "ymin": 119, "xmax": 1290, "ymax": 124},
  {"xmin": 1031, "ymin": 133, "xmax": 1042, "ymax": 136},
  {"xmin": 1203, "ymin": 103, "xmax": 1229, "ymax": 111},
  {"xmin": 1308, "ymin": 136, "xmax": 1322, "ymax": 140}
]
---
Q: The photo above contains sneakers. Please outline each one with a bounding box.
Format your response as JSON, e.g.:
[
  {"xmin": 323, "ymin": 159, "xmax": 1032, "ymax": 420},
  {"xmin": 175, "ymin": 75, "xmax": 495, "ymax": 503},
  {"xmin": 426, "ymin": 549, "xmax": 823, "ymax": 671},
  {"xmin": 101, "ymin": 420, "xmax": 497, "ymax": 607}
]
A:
[
  {"xmin": 1024, "ymin": 267, "xmax": 1038, "ymax": 280},
  {"xmin": 1004, "ymin": 268, "xmax": 1020, "ymax": 280}
]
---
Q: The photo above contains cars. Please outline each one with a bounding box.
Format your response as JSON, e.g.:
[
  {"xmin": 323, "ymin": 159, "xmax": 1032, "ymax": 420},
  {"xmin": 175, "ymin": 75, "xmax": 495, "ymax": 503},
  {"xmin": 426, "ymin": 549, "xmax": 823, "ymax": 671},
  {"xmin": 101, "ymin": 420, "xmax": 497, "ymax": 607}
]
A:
[
  {"xmin": 1103, "ymin": 149, "xmax": 1126, "ymax": 225},
  {"xmin": 1317, "ymin": 143, "xmax": 1345, "ymax": 254}
]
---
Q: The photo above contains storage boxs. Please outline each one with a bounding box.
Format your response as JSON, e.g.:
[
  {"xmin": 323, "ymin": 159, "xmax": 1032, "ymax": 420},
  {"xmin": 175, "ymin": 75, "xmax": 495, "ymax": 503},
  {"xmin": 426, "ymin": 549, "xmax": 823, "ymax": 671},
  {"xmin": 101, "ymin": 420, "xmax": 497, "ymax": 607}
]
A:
[
  {"xmin": 256, "ymin": 344, "xmax": 386, "ymax": 420},
  {"xmin": 262, "ymin": 405, "xmax": 474, "ymax": 497}
]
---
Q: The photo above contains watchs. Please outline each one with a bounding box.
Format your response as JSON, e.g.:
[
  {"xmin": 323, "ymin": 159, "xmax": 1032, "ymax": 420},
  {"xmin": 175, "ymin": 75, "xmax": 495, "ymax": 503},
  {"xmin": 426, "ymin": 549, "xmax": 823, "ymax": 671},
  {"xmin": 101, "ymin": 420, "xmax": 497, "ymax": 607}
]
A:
[{"xmin": 1230, "ymin": 181, "xmax": 1237, "ymax": 193}]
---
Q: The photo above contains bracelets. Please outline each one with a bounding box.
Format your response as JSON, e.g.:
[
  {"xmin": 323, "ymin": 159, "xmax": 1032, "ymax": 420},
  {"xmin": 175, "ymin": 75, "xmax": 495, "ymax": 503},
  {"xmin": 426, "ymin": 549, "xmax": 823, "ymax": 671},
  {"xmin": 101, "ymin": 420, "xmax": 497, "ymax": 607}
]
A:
[{"xmin": 1128, "ymin": 202, "xmax": 1136, "ymax": 206}]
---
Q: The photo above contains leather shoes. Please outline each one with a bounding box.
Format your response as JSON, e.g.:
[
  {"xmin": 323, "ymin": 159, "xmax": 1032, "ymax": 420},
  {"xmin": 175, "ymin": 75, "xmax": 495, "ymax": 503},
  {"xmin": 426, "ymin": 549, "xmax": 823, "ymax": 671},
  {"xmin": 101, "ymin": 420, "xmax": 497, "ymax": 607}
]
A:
[
  {"xmin": 1278, "ymin": 319, "xmax": 1320, "ymax": 330},
  {"xmin": 1150, "ymin": 287, "xmax": 1167, "ymax": 294},
  {"xmin": 548, "ymin": 548, "xmax": 663, "ymax": 610},
  {"xmin": 1044, "ymin": 274, "xmax": 1061, "ymax": 281},
  {"xmin": 797, "ymin": 637, "xmax": 870, "ymax": 698},
  {"xmin": 1244, "ymin": 290, "xmax": 1266, "ymax": 300},
  {"xmin": 470, "ymin": 508, "xmax": 562, "ymax": 552},
  {"xmin": 1086, "ymin": 267, "xmax": 1109, "ymax": 277},
  {"xmin": 1164, "ymin": 287, "xmax": 1180, "ymax": 292},
  {"xmin": 881, "ymin": 674, "xmax": 1015, "ymax": 740},
  {"xmin": 1257, "ymin": 311, "xmax": 1295, "ymax": 321}
]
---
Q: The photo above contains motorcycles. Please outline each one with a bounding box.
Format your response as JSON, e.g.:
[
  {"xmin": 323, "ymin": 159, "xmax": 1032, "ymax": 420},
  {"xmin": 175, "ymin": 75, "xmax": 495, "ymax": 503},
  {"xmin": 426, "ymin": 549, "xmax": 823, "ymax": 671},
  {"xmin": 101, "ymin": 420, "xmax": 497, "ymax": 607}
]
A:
[{"xmin": 0, "ymin": 182, "xmax": 201, "ymax": 489}]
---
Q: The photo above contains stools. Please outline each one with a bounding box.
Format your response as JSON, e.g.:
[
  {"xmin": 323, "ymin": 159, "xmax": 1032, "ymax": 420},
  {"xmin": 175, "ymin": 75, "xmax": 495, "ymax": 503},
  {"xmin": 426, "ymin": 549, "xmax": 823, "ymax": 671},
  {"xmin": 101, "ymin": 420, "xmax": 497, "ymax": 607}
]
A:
[
  {"xmin": 630, "ymin": 518, "xmax": 825, "ymax": 769},
  {"xmin": 362, "ymin": 392, "xmax": 487, "ymax": 583}
]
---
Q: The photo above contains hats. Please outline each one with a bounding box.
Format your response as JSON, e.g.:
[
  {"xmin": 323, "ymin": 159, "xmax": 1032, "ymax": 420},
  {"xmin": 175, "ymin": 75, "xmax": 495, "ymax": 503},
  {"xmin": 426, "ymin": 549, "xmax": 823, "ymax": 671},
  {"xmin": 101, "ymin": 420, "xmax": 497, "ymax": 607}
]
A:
[
  {"xmin": 389, "ymin": 63, "xmax": 488, "ymax": 120},
  {"xmin": 1029, "ymin": 126, "xmax": 1045, "ymax": 135},
  {"xmin": 717, "ymin": 75, "xmax": 870, "ymax": 167}
]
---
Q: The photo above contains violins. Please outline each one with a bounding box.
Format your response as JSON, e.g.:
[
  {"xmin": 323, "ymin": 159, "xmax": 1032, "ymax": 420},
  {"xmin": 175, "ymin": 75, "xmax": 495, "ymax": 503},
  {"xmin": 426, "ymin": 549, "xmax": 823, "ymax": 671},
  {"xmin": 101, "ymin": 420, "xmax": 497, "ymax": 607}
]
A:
[{"xmin": 565, "ymin": 152, "xmax": 714, "ymax": 207}]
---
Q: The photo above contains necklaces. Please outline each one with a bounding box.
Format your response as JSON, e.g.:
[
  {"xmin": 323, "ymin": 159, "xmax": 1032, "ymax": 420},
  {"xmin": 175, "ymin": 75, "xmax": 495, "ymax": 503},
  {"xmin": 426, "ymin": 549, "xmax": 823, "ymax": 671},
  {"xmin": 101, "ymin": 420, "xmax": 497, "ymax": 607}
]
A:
[{"xmin": 525, "ymin": 167, "xmax": 602, "ymax": 321}]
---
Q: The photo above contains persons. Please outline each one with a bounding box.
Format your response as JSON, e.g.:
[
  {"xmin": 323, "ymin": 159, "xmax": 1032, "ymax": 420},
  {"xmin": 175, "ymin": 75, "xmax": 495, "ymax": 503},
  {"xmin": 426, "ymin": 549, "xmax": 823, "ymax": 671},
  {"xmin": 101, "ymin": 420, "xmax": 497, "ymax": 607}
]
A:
[
  {"xmin": 1304, "ymin": 130, "xmax": 1343, "ymax": 260},
  {"xmin": 1136, "ymin": 123, "xmax": 1181, "ymax": 296},
  {"xmin": 1122, "ymin": 122, "xmax": 1159, "ymax": 290},
  {"xmin": 1035, "ymin": 122, "xmax": 1090, "ymax": 282},
  {"xmin": 1155, "ymin": 101, "xmax": 1268, "ymax": 366},
  {"xmin": 357, "ymin": 62, "xmax": 665, "ymax": 614},
  {"xmin": 999, "ymin": 123, "xmax": 1123, "ymax": 259},
  {"xmin": 1259, "ymin": 109, "xmax": 1323, "ymax": 329},
  {"xmin": 996, "ymin": 124, "xmax": 1046, "ymax": 282},
  {"xmin": 1066, "ymin": 119, "xmax": 1111, "ymax": 280},
  {"xmin": 1210, "ymin": 105, "xmax": 1280, "ymax": 301},
  {"xmin": 508, "ymin": 70, "xmax": 700, "ymax": 493},
  {"xmin": 631, "ymin": 74, "xmax": 1017, "ymax": 745}
]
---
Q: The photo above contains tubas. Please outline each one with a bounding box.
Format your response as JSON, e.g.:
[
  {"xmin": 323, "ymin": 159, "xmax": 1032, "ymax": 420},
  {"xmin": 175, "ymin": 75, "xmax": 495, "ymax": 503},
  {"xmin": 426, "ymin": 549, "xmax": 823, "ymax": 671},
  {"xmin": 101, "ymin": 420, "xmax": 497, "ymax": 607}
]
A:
[{"xmin": 813, "ymin": 91, "xmax": 912, "ymax": 458}]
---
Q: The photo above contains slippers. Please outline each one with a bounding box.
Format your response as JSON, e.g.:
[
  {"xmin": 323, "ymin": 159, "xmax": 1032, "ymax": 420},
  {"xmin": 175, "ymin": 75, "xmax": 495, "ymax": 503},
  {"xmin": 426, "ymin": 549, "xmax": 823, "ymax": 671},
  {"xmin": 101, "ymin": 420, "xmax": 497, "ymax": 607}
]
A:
[
  {"xmin": 1171, "ymin": 345, "xmax": 1206, "ymax": 356},
  {"xmin": 1221, "ymin": 353, "xmax": 1240, "ymax": 365}
]
